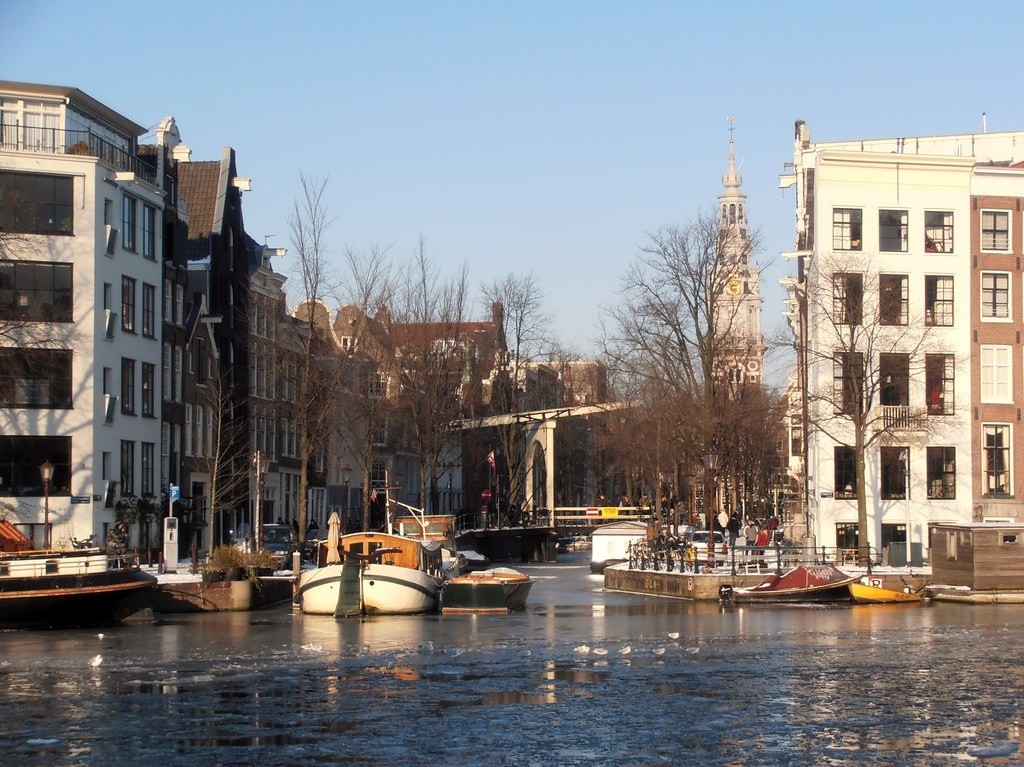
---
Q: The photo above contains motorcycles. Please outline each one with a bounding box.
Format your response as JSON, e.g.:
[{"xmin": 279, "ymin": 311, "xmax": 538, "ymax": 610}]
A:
[{"xmin": 229, "ymin": 523, "xmax": 253, "ymax": 554}]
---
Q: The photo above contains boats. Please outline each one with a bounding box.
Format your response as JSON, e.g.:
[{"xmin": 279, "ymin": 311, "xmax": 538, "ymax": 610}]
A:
[
  {"xmin": 298, "ymin": 529, "xmax": 443, "ymax": 617},
  {"xmin": 456, "ymin": 548, "xmax": 489, "ymax": 569},
  {"xmin": 718, "ymin": 562, "xmax": 862, "ymax": 603},
  {"xmin": 848, "ymin": 581, "xmax": 921, "ymax": 604},
  {"xmin": 440, "ymin": 566, "xmax": 534, "ymax": 614},
  {"xmin": 0, "ymin": 554, "xmax": 158, "ymax": 629}
]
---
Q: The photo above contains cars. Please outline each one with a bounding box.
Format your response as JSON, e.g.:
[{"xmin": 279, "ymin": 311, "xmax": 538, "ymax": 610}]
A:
[
  {"xmin": 688, "ymin": 530, "xmax": 729, "ymax": 566},
  {"xmin": 302, "ymin": 528, "xmax": 344, "ymax": 565},
  {"xmin": 667, "ymin": 525, "xmax": 696, "ymax": 547},
  {"xmin": 255, "ymin": 525, "xmax": 293, "ymax": 564}
]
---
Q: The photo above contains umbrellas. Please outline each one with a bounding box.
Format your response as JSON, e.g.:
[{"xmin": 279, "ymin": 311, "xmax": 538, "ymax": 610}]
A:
[{"xmin": 326, "ymin": 512, "xmax": 340, "ymax": 565}]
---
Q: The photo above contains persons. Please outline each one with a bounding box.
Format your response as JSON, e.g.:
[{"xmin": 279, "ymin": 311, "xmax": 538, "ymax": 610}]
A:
[
  {"xmin": 597, "ymin": 495, "xmax": 610, "ymax": 524},
  {"xmin": 622, "ymin": 496, "xmax": 631, "ymax": 521},
  {"xmin": 713, "ymin": 509, "xmax": 743, "ymax": 551},
  {"xmin": 647, "ymin": 517, "xmax": 677, "ymax": 572},
  {"xmin": 639, "ymin": 494, "xmax": 649, "ymax": 523},
  {"xmin": 276, "ymin": 517, "xmax": 285, "ymax": 525},
  {"xmin": 744, "ymin": 518, "xmax": 768, "ymax": 568},
  {"xmin": 309, "ymin": 519, "xmax": 320, "ymax": 530},
  {"xmin": 285, "ymin": 518, "xmax": 299, "ymax": 534}
]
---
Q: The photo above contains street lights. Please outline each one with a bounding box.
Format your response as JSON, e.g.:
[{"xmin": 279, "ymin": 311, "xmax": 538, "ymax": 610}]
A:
[
  {"xmin": 255, "ymin": 454, "xmax": 271, "ymax": 548},
  {"xmin": 39, "ymin": 460, "xmax": 55, "ymax": 549},
  {"xmin": 701, "ymin": 448, "xmax": 720, "ymax": 568},
  {"xmin": 341, "ymin": 463, "xmax": 353, "ymax": 530}
]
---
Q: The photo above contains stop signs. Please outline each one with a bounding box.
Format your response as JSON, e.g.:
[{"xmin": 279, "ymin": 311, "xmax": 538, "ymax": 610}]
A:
[{"xmin": 482, "ymin": 490, "xmax": 491, "ymax": 499}]
